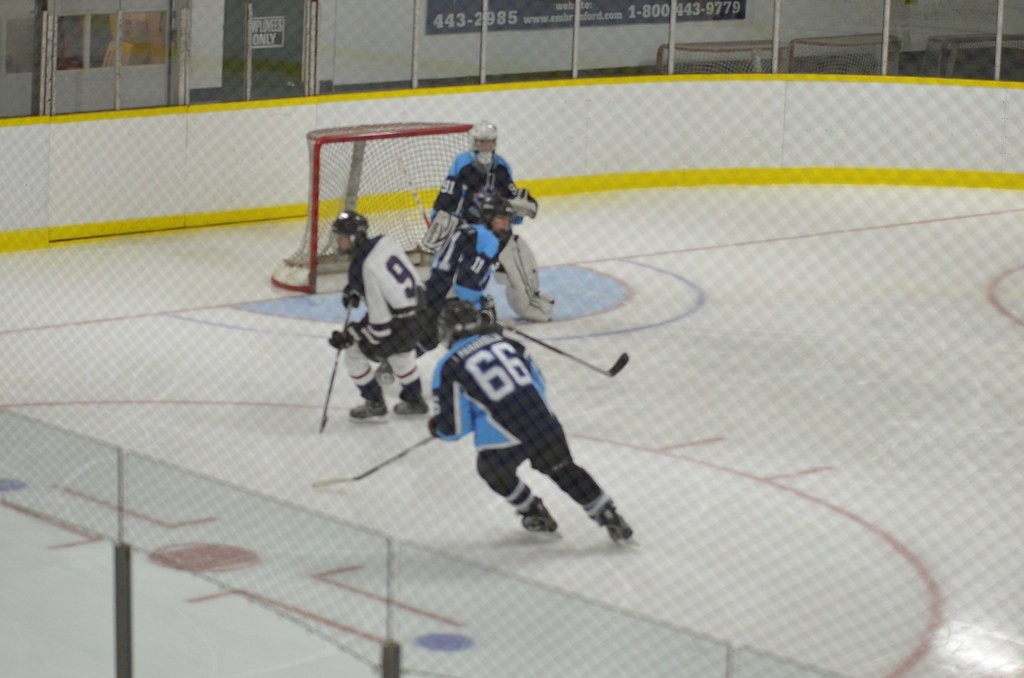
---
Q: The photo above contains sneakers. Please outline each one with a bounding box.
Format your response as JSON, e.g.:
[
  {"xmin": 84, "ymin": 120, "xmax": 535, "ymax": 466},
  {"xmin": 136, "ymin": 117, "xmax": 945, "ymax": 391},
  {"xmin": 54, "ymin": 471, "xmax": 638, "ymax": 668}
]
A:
[
  {"xmin": 595, "ymin": 501, "xmax": 641, "ymax": 547},
  {"xmin": 346, "ymin": 386, "xmax": 391, "ymax": 422},
  {"xmin": 391, "ymin": 385, "xmax": 433, "ymax": 419},
  {"xmin": 515, "ymin": 496, "xmax": 565, "ymax": 539}
]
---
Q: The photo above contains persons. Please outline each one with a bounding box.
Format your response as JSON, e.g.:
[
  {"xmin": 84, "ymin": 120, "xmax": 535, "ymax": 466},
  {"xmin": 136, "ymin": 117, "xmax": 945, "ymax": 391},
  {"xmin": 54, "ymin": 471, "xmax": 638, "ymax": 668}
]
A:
[
  {"xmin": 427, "ymin": 302, "xmax": 632, "ymax": 542},
  {"xmin": 328, "ymin": 212, "xmax": 428, "ymax": 418},
  {"xmin": 375, "ymin": 123, "xmax": 558, "ymax": 386}
]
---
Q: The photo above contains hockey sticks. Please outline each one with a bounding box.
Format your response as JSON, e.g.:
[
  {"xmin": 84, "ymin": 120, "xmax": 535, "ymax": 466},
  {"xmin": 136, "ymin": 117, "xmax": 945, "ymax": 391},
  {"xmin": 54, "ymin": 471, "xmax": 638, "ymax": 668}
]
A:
[
  {"xmin": 402, "ymin": 167, "xmax": 432, "ymax": 229},
  {"xmin": 318, "ymin": 299, "xmax": 354, "ymax": 434},
  {"xmin": 497, "ymin": 323, "xmax": 631, "ymax": 378}
]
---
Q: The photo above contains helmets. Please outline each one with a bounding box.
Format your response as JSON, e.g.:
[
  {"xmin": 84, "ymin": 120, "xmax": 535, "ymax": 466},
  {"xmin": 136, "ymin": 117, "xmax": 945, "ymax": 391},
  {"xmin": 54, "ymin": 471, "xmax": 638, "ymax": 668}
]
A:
[
  {"xmin": 440, "ymin": 297, "xmax": 482, "ymax": 335},
  {"xmin": 330, "ymin": 210, "xmax": 369, "ymax": 233},
  {"xmin": 469, "ymin": 119, "xmax": 497, "ymax": 140},
  {"xmin": 481, "ymin": 194, "xmax": 518, "ymax": 215}
]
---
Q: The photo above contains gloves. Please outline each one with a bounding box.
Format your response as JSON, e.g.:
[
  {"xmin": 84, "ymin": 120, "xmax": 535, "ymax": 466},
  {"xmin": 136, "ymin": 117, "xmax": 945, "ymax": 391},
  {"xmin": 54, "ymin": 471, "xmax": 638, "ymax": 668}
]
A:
[
  {"xmin": 426, "ymin": 414, "xmax": 438, "ymax": 438},
  {"xmin": 328, "ymin": 322, "xmax": 365, "ymax": 350},
  {"xmin": 341, "ymin": 284, "xmax": 360, "ymax": 308}
]
